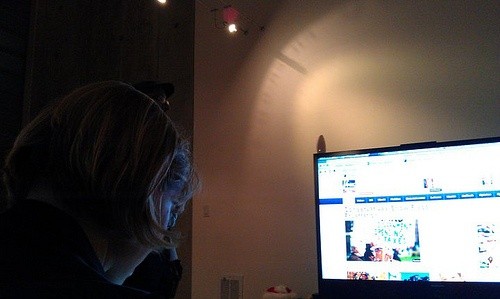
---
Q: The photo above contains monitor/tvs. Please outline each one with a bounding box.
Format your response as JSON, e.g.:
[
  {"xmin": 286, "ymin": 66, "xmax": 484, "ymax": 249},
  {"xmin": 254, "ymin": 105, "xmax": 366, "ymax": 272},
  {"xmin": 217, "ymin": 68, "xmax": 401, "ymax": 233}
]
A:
[{"xmin": 313, "ymin": 137, "xmax": 500, "ymax": 293}]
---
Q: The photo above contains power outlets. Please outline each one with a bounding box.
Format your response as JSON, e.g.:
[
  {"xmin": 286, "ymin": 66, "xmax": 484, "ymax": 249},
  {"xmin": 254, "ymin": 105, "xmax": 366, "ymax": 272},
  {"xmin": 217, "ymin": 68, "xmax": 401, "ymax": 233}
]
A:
[{"xmin": 203, "ymin": 205, "xmax": 211, "ymax": 217}]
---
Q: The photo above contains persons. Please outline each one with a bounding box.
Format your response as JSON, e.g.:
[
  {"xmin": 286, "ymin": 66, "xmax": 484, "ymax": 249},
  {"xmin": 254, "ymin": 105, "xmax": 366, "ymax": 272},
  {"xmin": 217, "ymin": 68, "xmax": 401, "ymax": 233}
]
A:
[{"xmin": 0, "ymin": 81, "xmax": 223, "ymax": 299}]
